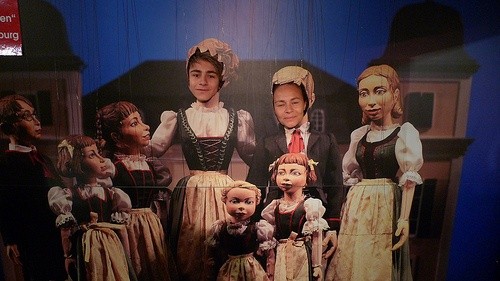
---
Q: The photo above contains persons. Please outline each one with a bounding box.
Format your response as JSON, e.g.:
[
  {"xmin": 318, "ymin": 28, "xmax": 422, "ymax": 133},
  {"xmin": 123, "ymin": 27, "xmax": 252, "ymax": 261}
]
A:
[
  {"xmin": 187, "ymin": 50, "xmax": 224, "ymax": 102},
  {"xmin": 273, "ymin": 83, "xmax": 309, "ymax": 129}
]
